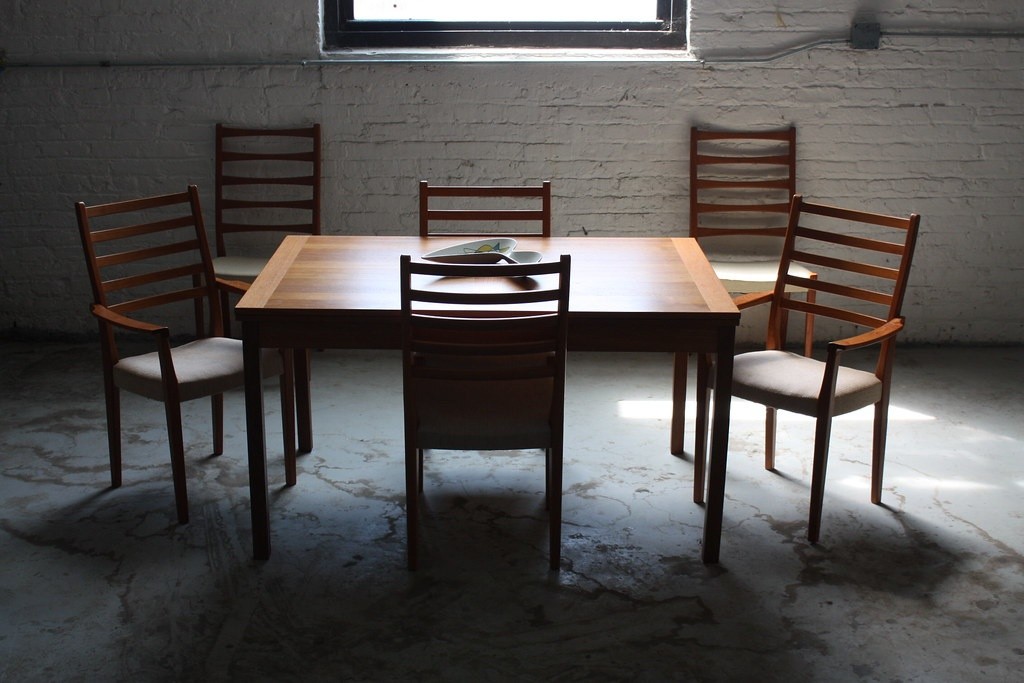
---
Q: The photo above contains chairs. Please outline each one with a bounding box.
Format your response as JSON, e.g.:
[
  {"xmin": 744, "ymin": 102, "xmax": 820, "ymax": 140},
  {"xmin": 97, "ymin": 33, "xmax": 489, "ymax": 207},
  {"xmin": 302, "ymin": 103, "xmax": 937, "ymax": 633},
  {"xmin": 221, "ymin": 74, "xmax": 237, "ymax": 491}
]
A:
[
  {"xmin": 73, "ymin": 184, "xmax": 297, "ymax": 526},
  {"xmin": 188, "ymin": 122, "xmax": 323, "ymax": 382},
  {"xmin": 400, "ymin": 179, "xmax": 571, "ymax": 570},
  {"xmin": 693, "ymin": 195, "xmax": 921, "ymax": 543},
  {"xmin": 689, "ymin": 125, "xmax": 817, "ymax": 369}
]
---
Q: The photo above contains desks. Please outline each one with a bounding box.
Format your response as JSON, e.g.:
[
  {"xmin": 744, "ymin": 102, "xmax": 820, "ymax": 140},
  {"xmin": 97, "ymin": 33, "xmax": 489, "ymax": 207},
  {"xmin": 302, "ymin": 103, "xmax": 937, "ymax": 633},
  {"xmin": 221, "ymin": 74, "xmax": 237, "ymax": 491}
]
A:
[{"xmin": 233, "ymin": 235, "xmax": 743, "ymax": 566}]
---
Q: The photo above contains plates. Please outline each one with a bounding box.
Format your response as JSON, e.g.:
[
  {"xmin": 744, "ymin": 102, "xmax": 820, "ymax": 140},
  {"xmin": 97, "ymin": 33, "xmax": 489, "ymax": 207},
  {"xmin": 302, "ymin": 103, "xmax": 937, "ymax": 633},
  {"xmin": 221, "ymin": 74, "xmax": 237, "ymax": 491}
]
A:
[{"xmin": 421, "ymin": 238, "xmax": 543, "ymax": 265}]
